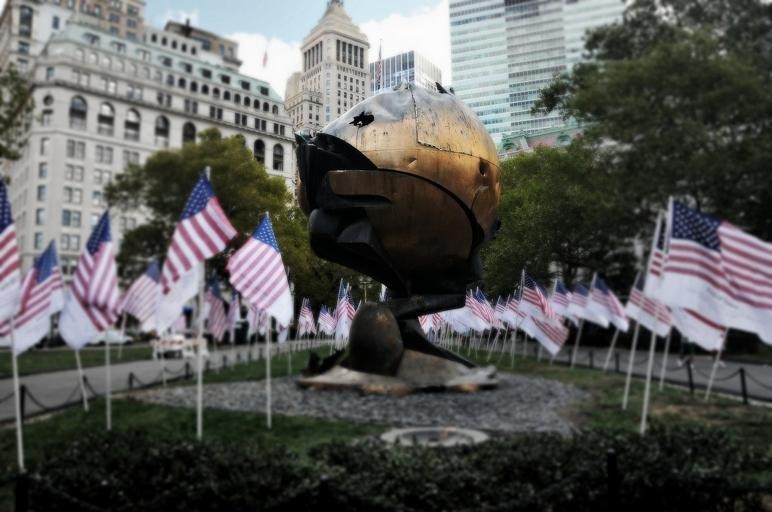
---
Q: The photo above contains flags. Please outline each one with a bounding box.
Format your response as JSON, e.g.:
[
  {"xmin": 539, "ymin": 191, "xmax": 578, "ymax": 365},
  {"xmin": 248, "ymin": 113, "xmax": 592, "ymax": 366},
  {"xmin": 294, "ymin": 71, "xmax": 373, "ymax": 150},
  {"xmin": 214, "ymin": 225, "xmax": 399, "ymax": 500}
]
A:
[
  {"xmin": 655, "ymin": 194, "xmax": 771, "ymax": 347},
  {"xmin": 588, "ymin": 273, "xmax": 630, "ymax": 332},
  {"xmin": 0, "ymin": 171, "xmax": 20, "ymax": 334},
  {"xmin": 113, "ymin": 248, "xmax": 170, "ymax": 334},
  {"xmin": 226, "ymin": 294, "xmax": 241, "ymax": 327},
  {"xmin": 152, "ymin": 162, "xmax": 239, "ymax": 337},
  {"xmin": 518, "ymin": 269, "xmax": 564, "ymax": 329},
  {"xmin": 277, "ymin": 321, "xmax": 290, "ymax": 342},
  {"xmin": 261, "ymin": 309, "xmax": 268, "ymax": 334},
  {"xmin": 417, "ymin": 287, "xmax": 517, "ymax": 335},
  {"xmin": 569, "ymin": 284, "xmax": 609, "ymax": 329},
  {"xmin": 246, "ymin": 305, "xmax": 258, "ymax": 339},
  {"xmin": 624, "ymin": 269, "xmax": 672, "ymax": 337},
  {"xmin": 645, "ymin": 208, "xmax": 715, "ymax": 350},
  {"xmin": 552, "ymin": 280, "xmax": 580, "ymax": 329},
  {"xmin": 227, "ymin": 207, "xmax": 295, "ymax": 328},
  {"xmin": 277, "ymin": 279, "xmax": 355, "ymax": 339},
  {"xmin": 203, "ymin": 279, "xmax": 229, "ymax": 341},
  {"xmin": 521, "ymin": 314, "xmax": 570, "ymax": 356},
  {"xmin": 55, "ymin": 206, "xmax": 125, "ymax": 350},
  {"xmin": 661, "ymin": 209, "xmax": 726, "ymax": 352},
  {"xmin": 11, "ymin": 238, "xmax": 63, "ymax": 357}
]
